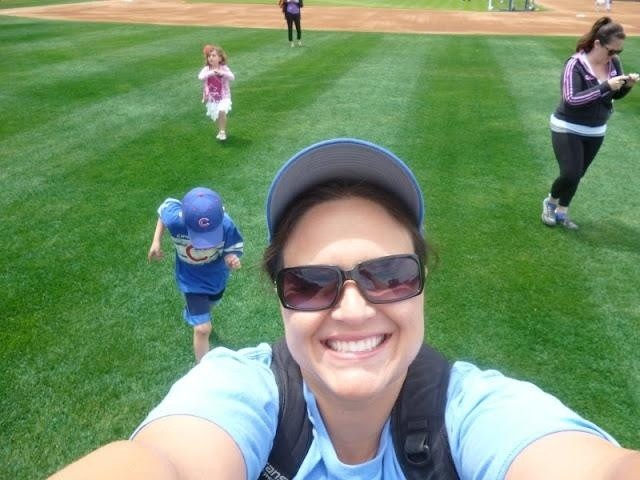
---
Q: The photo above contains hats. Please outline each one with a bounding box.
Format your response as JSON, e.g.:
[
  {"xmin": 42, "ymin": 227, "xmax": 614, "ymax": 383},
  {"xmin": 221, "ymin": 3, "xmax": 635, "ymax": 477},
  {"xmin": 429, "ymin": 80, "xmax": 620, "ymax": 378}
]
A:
[
  {"xmin": 266, "ymin": 138, "xmax": 424, "ymax": 244},
  {"xmin": 182, "ymin": 187, "xmax": 224, "ymax": 249}
]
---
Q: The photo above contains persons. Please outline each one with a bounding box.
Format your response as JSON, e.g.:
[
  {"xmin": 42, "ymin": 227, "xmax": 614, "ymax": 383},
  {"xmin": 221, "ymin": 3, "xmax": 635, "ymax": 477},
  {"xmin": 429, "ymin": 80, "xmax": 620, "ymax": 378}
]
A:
[
  {"xmin": 47, "ymin": 139, "xmax": 640, "ymax": 480},
  {"xmin": 283, "ymin": 0, "xmax": 303, "ymax": 48},
  {"xmin": 541, "ymin": 16, "xmax": 640, "ymax": 230},
  {"xmin": 198, "ymin": 45, "xmax": 235, "ymax": 141},
  {"xmin": 147, "ymin": 187, "xmax": 244, "ymax": 366}
]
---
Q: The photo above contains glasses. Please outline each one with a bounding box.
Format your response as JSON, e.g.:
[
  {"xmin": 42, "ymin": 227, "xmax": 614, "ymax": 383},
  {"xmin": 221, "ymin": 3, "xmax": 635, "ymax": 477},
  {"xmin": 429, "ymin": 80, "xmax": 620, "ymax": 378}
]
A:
[
  {"xmin": 276, "ymin": 255, "xmax": 425, "ymax": 311},
  {"xmin": 604, "ymin": 45, "xmax": 623, "ymax": 56}
]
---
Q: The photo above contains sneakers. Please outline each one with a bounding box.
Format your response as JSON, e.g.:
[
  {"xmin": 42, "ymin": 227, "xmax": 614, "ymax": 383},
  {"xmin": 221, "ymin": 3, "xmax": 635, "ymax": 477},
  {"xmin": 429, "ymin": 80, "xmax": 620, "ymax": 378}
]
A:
[
  {"xmin": 542, "ymin": 198, "xmax": 579, "ymax": 230},
  {"xmin": 216, "ymin": 130, "xmax": 226, "ymax": 140}
]
